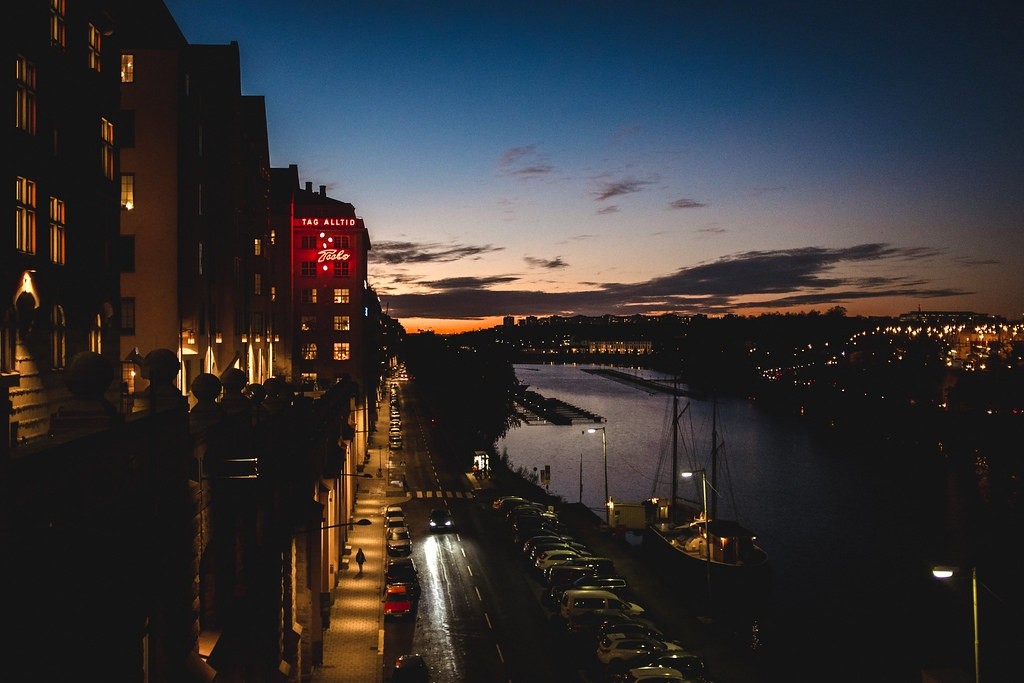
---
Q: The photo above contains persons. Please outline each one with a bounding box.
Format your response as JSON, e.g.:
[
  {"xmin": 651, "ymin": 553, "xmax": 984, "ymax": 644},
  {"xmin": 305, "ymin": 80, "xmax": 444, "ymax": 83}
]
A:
[{"xmin": 356, "ymin": 548, "xmax": 366, "ymax": 573}]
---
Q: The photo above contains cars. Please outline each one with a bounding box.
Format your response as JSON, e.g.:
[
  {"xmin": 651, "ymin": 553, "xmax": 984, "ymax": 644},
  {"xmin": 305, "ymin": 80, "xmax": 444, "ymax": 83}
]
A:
[
  {"xmin": 429, "ymin": 508, "xmax": 452, "ymax": 533},
  {"xmin": 383, "ymin": 585, "xmax": 414, "ymax": 618},
  {"xmin": 389, "ymin": 382, "xmax": 402, "ymax": 448},
  {"xmin": 386, "ymin": 506, "xmax": 405, "ymax": 526},
  {"xmin": 492, "ymin": 494, "xmax": 706, "ymax": 683},
  {"xmin": 384, "ymin": 558, "xmax": 416, "ymax": 582},
  {"xmin": 387, "ymin": 526, "xmax": 411, "ymax": 557},
  {"xmin": 392, "ymin": 654, "xmax": 428, "ymax": 682}
]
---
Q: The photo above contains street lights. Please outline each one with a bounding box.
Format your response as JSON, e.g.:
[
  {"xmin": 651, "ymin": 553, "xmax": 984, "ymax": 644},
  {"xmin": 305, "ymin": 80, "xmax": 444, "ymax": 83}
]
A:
[
  {"xmin": 679, "ymin": 470, "xmax": 713, "ymax": 620},
  {"xmin": 932, "ymin": 564, "xmax": 981, "ymax": 683},
  {"xmin": 587, "ymin": 428, "xmax": 609, "ymax": 541}
]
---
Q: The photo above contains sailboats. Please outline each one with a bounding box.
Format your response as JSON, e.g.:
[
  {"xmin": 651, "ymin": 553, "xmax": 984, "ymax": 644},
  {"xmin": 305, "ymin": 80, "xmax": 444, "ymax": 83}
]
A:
[{"xmin": 643, "ymin": 374, "xmax": 770, "ymax": 576}]
